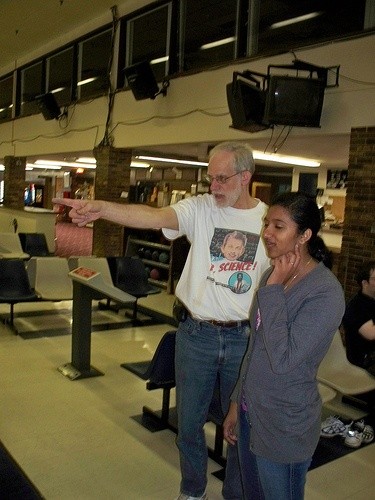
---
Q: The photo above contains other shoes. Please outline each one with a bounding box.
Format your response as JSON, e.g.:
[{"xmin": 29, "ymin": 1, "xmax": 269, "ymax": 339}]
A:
[{"xmin": 177, "ymin": 493, "xmax": 208, "ymax": 500}]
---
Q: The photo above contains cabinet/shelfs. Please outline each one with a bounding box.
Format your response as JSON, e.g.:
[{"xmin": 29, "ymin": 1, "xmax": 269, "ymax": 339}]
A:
[{"xmin": 124, "ymin": 235, "xmax": 190, "ymax": 295}]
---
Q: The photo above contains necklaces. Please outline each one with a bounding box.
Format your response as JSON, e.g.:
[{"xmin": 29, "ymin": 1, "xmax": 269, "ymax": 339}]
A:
[{"xmin": 284, "ymin": 257, "xmax": 313, "ymax": 291}]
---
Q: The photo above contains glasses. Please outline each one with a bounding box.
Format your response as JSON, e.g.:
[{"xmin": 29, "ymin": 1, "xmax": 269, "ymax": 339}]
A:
[{"xmin": 203, "ymin": 171, "xmax": 241, "ymax": 184}]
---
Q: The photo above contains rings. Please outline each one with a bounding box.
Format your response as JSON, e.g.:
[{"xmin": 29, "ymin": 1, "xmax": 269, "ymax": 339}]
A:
[{"xmin": 288, "ymin": 262, "xmax": 295, "ymax": 264}]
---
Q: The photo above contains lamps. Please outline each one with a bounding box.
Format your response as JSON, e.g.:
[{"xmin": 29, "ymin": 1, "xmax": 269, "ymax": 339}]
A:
[{"xmin": 76, "ymin": 150, "xmax": 320, "ymax": 168}]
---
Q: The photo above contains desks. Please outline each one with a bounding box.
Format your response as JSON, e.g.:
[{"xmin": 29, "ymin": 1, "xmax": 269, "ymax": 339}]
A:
[{"xmin": 137, "ymin": 294, "xmax": 178, "ymax": 328}]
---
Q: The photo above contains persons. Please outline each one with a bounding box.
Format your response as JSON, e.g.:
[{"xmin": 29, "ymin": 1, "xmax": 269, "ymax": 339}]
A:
[
  {"xmin": 223, "ymin": 189, "xmax": 347, "ymax": 500},
  {"xmin": 51, "ymin": 142, "xmax": 269, "ymax": 500},
  {"xmin": 343, "ymin": 261, "xmax": 375, "ymax": 373}
]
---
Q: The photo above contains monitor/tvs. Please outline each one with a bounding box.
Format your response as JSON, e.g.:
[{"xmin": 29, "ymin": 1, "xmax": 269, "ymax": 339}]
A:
[
  {"xmin": 225, "ymin": 80, "xmax": 269, "ymax": 134},
  {"xmin": 262, "ymin": 76, "xmax": 326, "ymax": 129}
]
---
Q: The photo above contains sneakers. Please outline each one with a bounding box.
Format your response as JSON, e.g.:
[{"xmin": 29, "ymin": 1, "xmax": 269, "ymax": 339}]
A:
[
  {"xmin": 345, "ymin": 420, "xmax": 374, "ymax": 447},
  {"xmin": 320, "ymin": 415, "xmax": 353, "ymax": 437}
]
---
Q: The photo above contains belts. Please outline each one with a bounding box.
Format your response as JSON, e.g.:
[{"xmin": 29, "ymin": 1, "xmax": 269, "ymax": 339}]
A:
[{"xmin": 208, "ymin": 320, "xmax": 250, "ymax": 328}]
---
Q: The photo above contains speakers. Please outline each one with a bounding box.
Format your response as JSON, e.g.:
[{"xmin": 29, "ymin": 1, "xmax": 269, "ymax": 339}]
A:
[
  {"xmin": 124, "ymin": 61, "xmax": 159, "ymax": 100},
  {"xmin": 36, "ymin": 93, "xmax": 61, "ymax": 120}
]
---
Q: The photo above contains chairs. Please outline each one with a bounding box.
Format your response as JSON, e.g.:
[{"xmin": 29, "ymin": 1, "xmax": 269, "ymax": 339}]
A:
[
  {"xmin": 120, "ymin": 330, "xmax": 176, "ymax": 432},
  {"xmin": 0, "ymin": 232, "xmax": 161, "ymax": 336},
  {"xmin": 207, "ymin": 329, "xmax": 375, "ymax": 468}
]
